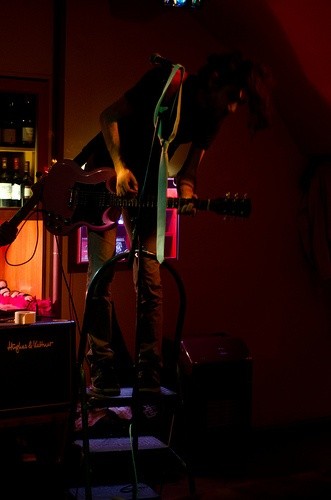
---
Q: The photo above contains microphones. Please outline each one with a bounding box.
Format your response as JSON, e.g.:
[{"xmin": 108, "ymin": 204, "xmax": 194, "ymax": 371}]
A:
[{"xmin": 151, "ymin": 53, "xmax": 183, "ymax": 70}]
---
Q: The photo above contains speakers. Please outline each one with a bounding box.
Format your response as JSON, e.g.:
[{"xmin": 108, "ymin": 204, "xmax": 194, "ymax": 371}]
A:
[
  {"xmin": 0, "ymin": 320, "xmax": 76, "ymax": 416},
  {"xmin": 163, "ymin": 330, "xmax": 255, "ymax": 474}
]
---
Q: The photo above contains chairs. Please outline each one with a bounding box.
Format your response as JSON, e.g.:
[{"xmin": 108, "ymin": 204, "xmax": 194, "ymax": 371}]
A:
[{"xmin": 63, "ymin": 251, "xmax": 194, "ymax": 500}]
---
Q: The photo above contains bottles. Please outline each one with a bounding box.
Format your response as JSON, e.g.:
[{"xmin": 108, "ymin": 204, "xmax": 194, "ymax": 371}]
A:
[
  {"xmin": 0, "ymin": 157, "xmax": 33, "ymax": 209},
  {"xmin": 0, "ymin": 92, "xmax": 36, "ymax": 148}
]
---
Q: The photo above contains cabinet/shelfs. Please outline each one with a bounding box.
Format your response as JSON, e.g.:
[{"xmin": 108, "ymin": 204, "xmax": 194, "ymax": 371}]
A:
[{"xmin": 0, "ymin": 76, "xmax": 53, "ymax": 300}]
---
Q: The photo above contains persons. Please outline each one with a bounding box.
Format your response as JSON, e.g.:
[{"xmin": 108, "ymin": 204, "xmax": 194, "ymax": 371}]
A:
[{"xmin": 85, "ymin": 49, "xmax": 272, "ymax": 394}]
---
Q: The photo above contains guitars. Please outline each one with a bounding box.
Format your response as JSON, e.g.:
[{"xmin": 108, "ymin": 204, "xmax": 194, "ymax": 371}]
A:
[
  {"xmin": 40, "ymin": 158, "xmax": 254, "ymax": 238},
  {"xmin": 0, "ymin": 176, "xmax": 45, "ymax": 247}
]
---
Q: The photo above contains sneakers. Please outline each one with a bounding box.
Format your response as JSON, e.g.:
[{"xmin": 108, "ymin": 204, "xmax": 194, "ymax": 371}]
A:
[
  {"xmin": 137, "ymin": 362, "xmax": 160, "ymax": 393},
  {"xmin": 91, "ymin": 365, "xmax": 120, "ymax": 393}
]
